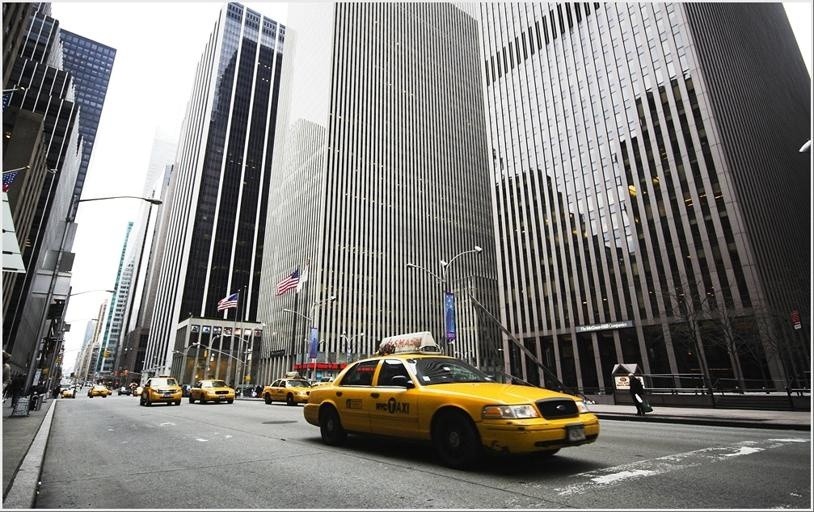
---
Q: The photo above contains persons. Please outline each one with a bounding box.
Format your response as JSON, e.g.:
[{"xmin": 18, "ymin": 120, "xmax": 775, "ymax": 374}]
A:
[
  {"xmin": 628, "ymin": 373, "xmax": 646, "ymax": 416},
  {"xmin": 235, "ymin": 384, "xmax": 264, "ymax": 399},
  {"xmin": 3, "ymin": 352, "xmax": 46, "ymax": 410},
  {"xmin": 80, "ymin": 383, "xmax": 82, "ymax": 390}
]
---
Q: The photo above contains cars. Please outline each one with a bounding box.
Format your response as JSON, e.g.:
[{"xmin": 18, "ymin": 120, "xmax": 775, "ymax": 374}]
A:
[
  {"xmin": 261, "ymin": 378, "xmax": 311, "ymax": 405},
  {"xmin": 87, "ymin": 385, "xmax": 143, "ymax": 399},
  {"xmin": 188, "ymin": 378, "xmax": 235, "ymax": 405},
  {"xmin": 139, "ymin": 376, "xmax": 182, "ymax": 407},
  {"xmin": 301, "ymin": 354, "xmax": 600, "ymax": 473},
  {"xmin": 59, "ymin": 386, "xmax": 76, "ymax": 398}
]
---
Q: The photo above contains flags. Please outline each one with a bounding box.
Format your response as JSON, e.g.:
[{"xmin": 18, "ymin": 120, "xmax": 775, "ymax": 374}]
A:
[
  {"xmin": 217, "ymin": 292, "xmax": 238, "ymax": 312},
  {"xmin": 276, "ymin": 265, "xmax": 308, "ymax": 296},
  {"xmin": 3, "ymin": 171, "xmax": 19, "ymax": 192}
]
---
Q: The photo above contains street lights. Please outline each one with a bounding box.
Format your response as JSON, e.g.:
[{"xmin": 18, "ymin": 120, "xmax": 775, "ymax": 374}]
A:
[
  {"xmin": 173, "ymin": 342, "xmax": 195, "ymax": 384},
  {"xmin": 40, "ymin": 285, "xmax": 118, "ymax": 403},
  {"xmin": 11, "ymin": 188, "xmax": 163, "ymax": 419},
  {"xmin": 223, "ymin": 323, "xmax": 268, "ymax": 399},
  {"xmin": 403, "ymin": 244, "xmax": 481, "ymax": 355},
  {"xmin": 281, "ymin": 296, "xmax": 365, "ymax": 381},
  {"xmin": 192, "ymin": 334, "xmax": 219, "ymax": 379}
]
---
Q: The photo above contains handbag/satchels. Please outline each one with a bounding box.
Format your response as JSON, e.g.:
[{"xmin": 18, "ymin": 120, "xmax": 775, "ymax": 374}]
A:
[{"xmin": 641, "ymin": 403, "xmax": 653, "ymax": 412}]
